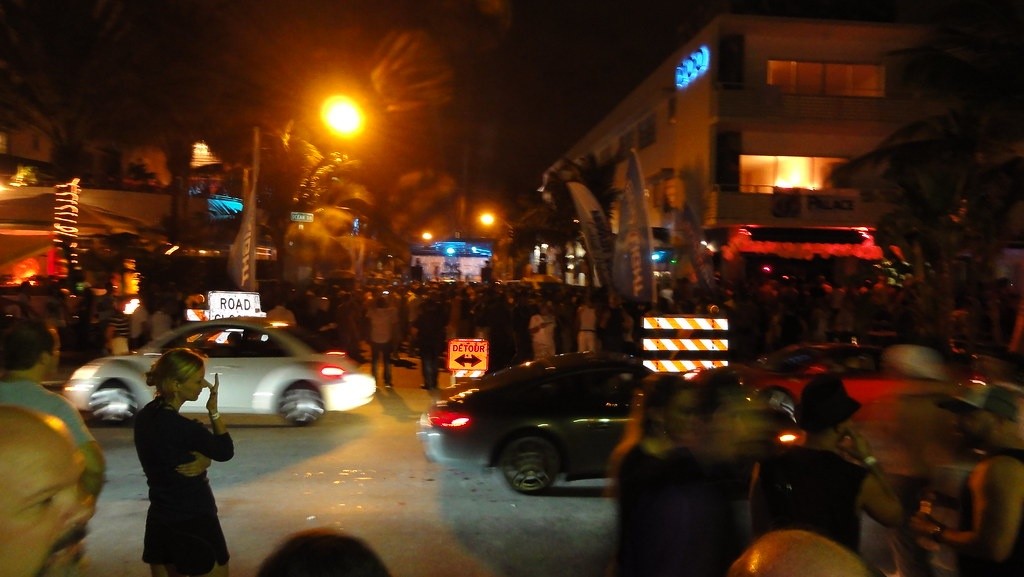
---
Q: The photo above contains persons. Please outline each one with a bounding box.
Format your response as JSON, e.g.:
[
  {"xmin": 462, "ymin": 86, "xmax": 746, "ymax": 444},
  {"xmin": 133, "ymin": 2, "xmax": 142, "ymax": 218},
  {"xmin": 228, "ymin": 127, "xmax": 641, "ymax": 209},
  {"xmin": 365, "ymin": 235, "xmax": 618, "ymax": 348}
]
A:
[
  {"xmin": 0, "ymin": 316, "xmax": 109, "ymax": 577},
  {"xmin": 609, "ymin": 339, "xmax": 1023, "ymax": 577},
  {"xmin": 133, "ymin": 348, "xmax": 235, "ymax": 577},
  {"xmin": 255, "ymin": 528, "xmax": 392, "ymax": 577},
  {"xmin": 0, "ymin": 257, "xmax": 1024, "ymax": 393}
]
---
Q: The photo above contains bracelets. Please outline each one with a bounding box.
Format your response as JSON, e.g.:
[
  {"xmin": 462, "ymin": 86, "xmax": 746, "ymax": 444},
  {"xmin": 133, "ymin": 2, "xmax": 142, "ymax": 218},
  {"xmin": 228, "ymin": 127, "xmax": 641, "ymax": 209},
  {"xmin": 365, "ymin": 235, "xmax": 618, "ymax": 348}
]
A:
[
  {"xmin": 933, "ymin": 524, "xmax": 945, "ymax": 537},
  {"xmin": 208, "ymin": 412, "xmax": 220, "ymax": 421}
]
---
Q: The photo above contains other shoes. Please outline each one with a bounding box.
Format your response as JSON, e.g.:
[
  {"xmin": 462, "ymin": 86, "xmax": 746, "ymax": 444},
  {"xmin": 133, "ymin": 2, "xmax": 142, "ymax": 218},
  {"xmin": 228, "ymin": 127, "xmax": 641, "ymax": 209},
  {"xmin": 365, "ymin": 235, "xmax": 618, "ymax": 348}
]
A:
[{"xmin": 421, "ymin": 382, "xmax": 433, "ymax": 390}]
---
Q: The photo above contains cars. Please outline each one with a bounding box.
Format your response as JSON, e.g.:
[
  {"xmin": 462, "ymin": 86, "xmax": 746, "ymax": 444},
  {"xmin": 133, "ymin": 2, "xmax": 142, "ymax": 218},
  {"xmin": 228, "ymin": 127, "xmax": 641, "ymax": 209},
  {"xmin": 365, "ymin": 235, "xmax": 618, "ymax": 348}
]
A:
[
  {"xmin": 59, "ymin": 317, "xmax": 377, "ymax": 425},
  {"xmin": 736, "ymin": 338, "xmax": 989, "ymax": 432},
  {"xmin": 413, "ymin": 351, "xmax": 741, "ymax": 497}
]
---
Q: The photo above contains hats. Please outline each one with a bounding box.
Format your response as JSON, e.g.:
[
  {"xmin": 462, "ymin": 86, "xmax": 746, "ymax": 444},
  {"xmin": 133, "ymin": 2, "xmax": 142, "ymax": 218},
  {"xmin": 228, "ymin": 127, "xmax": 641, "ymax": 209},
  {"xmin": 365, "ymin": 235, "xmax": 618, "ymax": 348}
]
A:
[
  {"xmin": 935, "ymin": 383, "xmax": 1024, "ymax": 424},
  {"xmin": 798, "ymin": 376, "xmax": 861, "ymax": 434}
]
